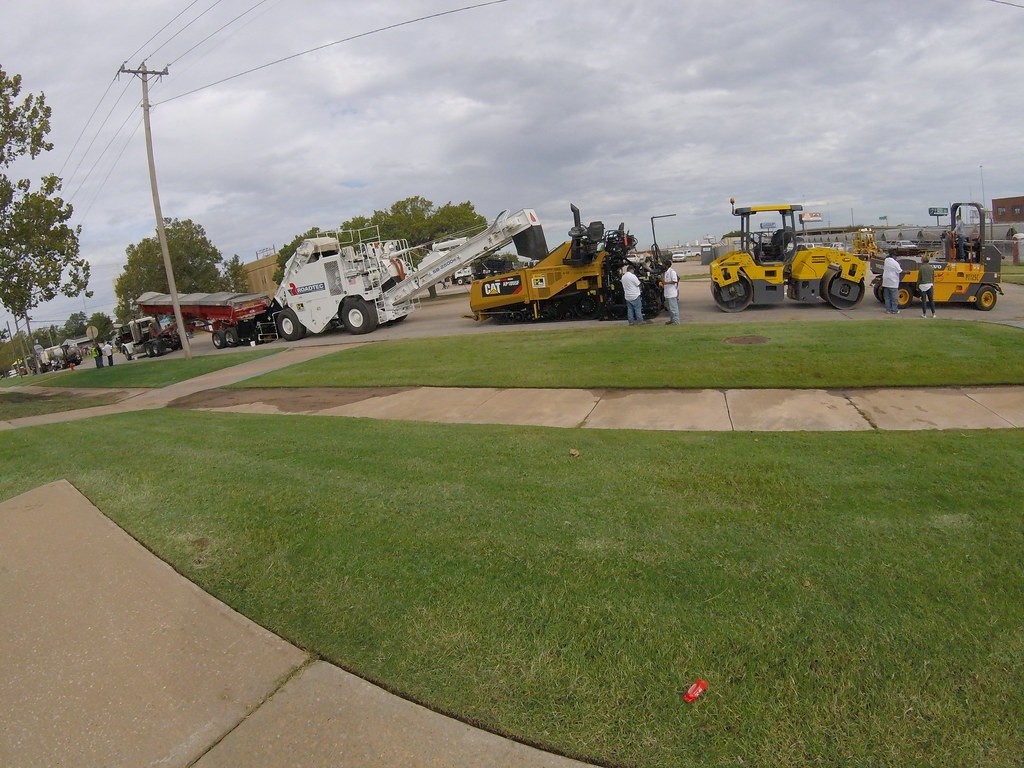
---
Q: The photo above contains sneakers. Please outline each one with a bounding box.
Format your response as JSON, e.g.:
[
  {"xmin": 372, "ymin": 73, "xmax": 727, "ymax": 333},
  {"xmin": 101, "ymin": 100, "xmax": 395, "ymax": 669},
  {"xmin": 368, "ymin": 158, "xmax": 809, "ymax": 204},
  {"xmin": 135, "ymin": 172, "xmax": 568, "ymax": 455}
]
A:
[
  {"xmin": 931, "ymin": 315, "xmax": 936, "ymax": 319},
  {"xmin": 665, "ymin": 320, "xmax": 681, "ymax": 326},
  {"xmin": 886, "ymin": 311, "xmax": 901, "ymax": 314},
  {"xmin": 628, "ymin": 321, "xmax": 648, "ymax": 325},
  {"xmin": 921, "ymin": 315, "xmax": 926, "ymax": 318}
]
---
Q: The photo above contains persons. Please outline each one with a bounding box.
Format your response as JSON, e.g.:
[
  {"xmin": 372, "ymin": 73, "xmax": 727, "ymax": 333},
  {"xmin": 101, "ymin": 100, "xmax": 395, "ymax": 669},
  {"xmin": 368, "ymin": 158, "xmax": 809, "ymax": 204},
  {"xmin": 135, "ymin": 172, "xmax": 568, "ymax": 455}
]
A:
[
  {"xmin": 882, "ymin": 252, "xmax": 910, "ymax": 314},
  {"xmin": 621, "ymin": 265, "xmax": 646, "ymax": 325},
  {"xmin": 115, "ymin": 337, "xmax": 122, "ymax": 353},
  {"xmin": 951, "ymin": 215, "xmax": 966, "ymax": 262},
  {"xmin": 659, "ymin": 260, "xmax": 680, "ymax": 325},
  {"xmin": 916, "ymin": 257, "xmax": 936, "ymax": 318},
  {"xmin": 51, "ymin": 358, "xmax": 56, "ymax": 372},
  {"xmin": 148, "ymin": 323, "xmax": 156, "ymax": 337},
  {"xmin": 90, "ymin": 342, "xmax": 114, "ymax": 367}
]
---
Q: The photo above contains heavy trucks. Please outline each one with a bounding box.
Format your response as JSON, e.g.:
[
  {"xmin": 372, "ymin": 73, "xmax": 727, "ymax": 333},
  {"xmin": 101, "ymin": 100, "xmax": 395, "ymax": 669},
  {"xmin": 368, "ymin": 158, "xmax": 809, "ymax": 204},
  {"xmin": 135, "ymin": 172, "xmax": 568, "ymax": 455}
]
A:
[{"xmin": 113, "ymin": 292, "xmax": 272, "ymax": 361}]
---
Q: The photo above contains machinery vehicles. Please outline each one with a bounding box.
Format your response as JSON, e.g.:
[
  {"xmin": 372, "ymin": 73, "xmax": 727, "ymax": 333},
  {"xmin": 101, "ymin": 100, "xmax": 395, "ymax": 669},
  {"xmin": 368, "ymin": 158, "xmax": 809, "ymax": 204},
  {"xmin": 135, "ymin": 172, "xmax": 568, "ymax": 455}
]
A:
[
  {"xmin": 461, "ymin": 203, "xmax": 680, "ymax": 321},
  {"xmin": 710, "ymin": 197, "xmax": 868, "ymax": 313},
  {"xmin": 267, "ymin": 208, "xmax": 549, "ymax": 341},
  {"xmin": 870, "ymin": 202, "xmax": 1004, "ymax": 311}
]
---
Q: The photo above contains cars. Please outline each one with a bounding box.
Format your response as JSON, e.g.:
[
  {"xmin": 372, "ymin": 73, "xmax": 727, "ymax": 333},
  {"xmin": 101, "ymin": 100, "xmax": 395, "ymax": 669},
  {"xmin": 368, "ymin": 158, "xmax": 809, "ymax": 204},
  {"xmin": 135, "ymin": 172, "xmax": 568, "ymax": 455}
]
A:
[
  {"xmin": 786, "ymin": 239, "xmax": 917, "ymax": 257},
  {"xmin": 622, "ymin": 249, "xmax": 701, "ymax": 266}
]
